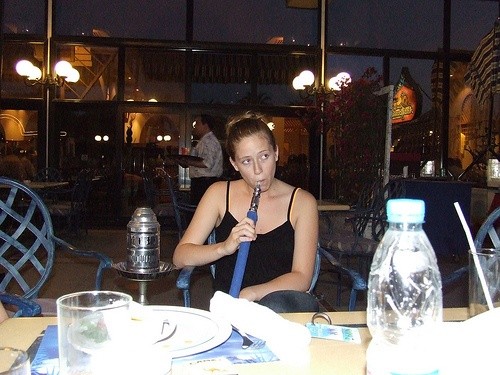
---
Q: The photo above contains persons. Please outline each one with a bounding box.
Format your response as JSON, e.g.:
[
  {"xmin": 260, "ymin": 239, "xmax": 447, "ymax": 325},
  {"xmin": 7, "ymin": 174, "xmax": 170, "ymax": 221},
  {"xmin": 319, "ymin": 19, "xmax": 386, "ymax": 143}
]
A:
[
  {"xmin": 280, "ymin": 154, "xmax": 313, "ymax": 187},
  {"xmin": 445, "ymin": 156, "xmax": 467, "ymax": 180},
  {"xmin": 0, "ymin": 145, "xmax": 41, "ymax": 182},
  {"xmin": 175, "ymin": 113, "xmax": 224, "ymax": 236},
  {"xmin": 328, "ymin": 130, "xmax": 374, "ymax": 194},
  {"xmin": 116, "ymin": 140, "xmax": 180, "ymax": 190},
  {"xmin": 170, "ymin": 117, "xmax": 320, "ymax": 313}
]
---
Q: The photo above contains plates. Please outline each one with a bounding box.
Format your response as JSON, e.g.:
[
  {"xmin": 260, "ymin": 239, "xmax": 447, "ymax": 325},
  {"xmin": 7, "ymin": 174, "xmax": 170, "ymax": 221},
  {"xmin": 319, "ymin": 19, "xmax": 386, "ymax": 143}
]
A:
[{"xmin": 68, "ymin": 305, "xmax": 232, "ymax": 359}]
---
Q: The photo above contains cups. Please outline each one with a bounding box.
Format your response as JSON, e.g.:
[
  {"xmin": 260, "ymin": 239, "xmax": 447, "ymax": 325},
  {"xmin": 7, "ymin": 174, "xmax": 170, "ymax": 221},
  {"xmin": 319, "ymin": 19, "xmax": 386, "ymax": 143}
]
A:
[
  {"xmin": 56, "ymin": 291, "xmax": 133, "ymax": 375},
  {"xmin": 390, "ymin": 166, "xmax": 415, "ymax": 180},
  {"xmin": 0, "ymin": 348, "xmax": 31, "ymax": 375},
  {"xmin": 166, "ymin": 145, "xmax": 198, "ymax": 157},
  {"xmin": 469, "ymin": 249, "xmax": 500, "ymax": 318}
]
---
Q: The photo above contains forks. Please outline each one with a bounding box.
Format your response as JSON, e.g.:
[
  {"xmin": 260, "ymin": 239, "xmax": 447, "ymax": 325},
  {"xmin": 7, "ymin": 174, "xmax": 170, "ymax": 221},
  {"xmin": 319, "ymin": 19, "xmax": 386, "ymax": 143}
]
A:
[{"xmin": 232, "ymin": 326, "xmax": 266, "ymax": 350}]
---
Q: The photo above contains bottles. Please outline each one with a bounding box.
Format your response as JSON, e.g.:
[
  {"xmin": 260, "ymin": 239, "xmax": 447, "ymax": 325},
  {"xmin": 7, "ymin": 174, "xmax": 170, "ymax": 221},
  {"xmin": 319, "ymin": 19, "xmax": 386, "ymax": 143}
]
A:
[{"xmin": 366, "ymin": 200, "xmax": 442, "ymax": 375}]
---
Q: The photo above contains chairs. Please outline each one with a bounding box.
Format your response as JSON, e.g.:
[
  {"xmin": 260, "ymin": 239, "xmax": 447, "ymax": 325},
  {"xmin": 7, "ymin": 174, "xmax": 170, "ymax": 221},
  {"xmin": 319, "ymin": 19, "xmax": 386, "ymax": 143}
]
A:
[
  {"xmin": 176, "ymin": 227, "xmax": 367, "ymax": 311},
  {"xmin": 61, "ymin": 171, "xmax": 92, "ymax": 240},
  {"xmin": 442, "ymin": 206, "xmax": 500, "ymax": 307},
  {"xmin": 0, "ymin": 179, "xmax": 111, "ymax": 317},
  {"xmin": 318, "ymin": 177, "xmax": 406, "ymax": 306}
]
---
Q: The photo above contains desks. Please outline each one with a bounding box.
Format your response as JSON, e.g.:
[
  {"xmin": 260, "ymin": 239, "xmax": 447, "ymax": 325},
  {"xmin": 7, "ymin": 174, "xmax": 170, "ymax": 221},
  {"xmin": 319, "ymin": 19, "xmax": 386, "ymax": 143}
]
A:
[
  {"xmin": 0, "ymin": 182, "xmax": 69, "ymax": 232},
  {"xmin": 0, "ymin": 307, "xmax": 468, "ymax": 375},
  {"xmin": 318, "ymin": 205, "xmax": 355, "ymax": 284}
]
